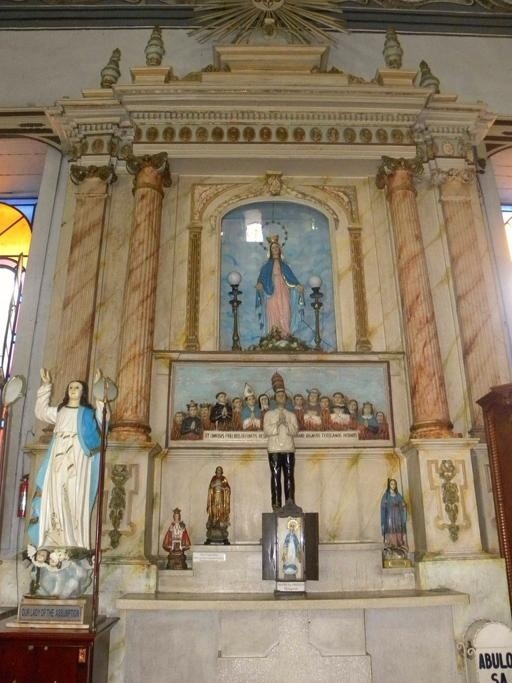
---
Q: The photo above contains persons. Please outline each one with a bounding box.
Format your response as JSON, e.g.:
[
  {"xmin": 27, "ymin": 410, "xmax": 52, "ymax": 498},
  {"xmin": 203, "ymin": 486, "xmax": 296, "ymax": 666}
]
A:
[
  {"xmin": 207, "ymin": 466, "xmax": 231, "ymax": 520},
  {"xmin": 163, "ymin": 509, "xmax": 191, "ymax": 552},
  {"xmin": 255, "ymin": 243, "xmax": 309, "ymax": 332},
  {"xmin": 281, "ymin": 520, "xmax": 303, "ymax": 574},
  {"xmin": 35, "ymin": 550, "xmax": 50, "ymax": 563},
  {"xmin": 34, "ymin": 366, "xmax": 112, "ymax": 551},
  {"xmin": 380, "ymin": 480, "xmax": 407, "ymax": 548},
  {"xmin": 172, "ymin": 372, "xmax": 389, "ymax": 439},
  {"xmin": 263, "ymin": 388, "xmax": 303, "ymax": 513}
]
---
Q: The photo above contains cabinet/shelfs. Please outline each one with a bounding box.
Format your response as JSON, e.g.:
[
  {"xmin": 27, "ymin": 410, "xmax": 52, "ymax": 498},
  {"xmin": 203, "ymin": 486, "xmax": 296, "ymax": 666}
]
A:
[{"xmin": 0, "ymin": 614, "xmax": 120, "ymax": 683}]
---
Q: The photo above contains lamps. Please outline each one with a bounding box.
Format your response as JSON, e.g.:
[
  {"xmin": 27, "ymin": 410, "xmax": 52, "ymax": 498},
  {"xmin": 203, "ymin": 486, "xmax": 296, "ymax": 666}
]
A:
[
  {"xmin": 227, "ymin": 271, "xmax": 244, "ymax": 350},
  {"xmin": 308, "ymin": 276, "xmax": 324, "ymax": 351}
]
